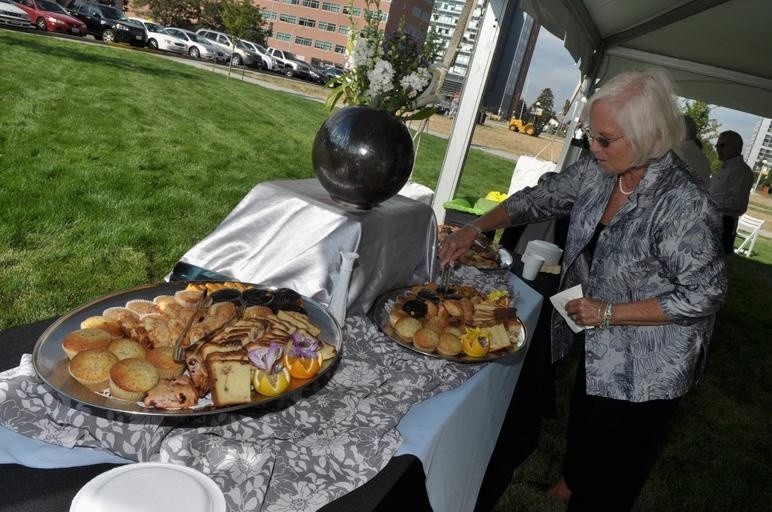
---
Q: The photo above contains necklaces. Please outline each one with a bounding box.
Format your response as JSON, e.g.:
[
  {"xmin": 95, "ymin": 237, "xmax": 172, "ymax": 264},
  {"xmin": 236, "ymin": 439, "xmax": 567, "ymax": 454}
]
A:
[{"xmin": 617, "ymin": 175, "xmax": 635, "ymax": 195}]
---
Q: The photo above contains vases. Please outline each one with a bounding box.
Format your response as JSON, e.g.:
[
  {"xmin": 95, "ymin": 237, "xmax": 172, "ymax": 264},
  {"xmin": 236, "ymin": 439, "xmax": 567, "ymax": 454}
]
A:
[{"xmin": 311, "ymin": 106, "xmax": 415, "ymax": 213}]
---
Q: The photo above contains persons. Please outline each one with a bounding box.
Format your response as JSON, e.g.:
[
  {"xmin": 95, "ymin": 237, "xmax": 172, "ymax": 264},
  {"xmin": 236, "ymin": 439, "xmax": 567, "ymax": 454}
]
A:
[
  {"xmin": 673, "ymin": 115, "xmax": 712, "ymax": 185},
  {"xmin": 435, "ymin": 70, "xmax": 730, "ymax": 512},
  {"xmin": 708, "ymin": 130, "xmax": 753, "ymax": 257}
]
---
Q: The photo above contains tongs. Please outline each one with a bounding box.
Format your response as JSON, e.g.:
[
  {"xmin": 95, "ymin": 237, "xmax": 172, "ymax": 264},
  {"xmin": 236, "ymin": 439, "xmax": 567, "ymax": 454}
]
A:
[{"xmin": 439, "ymin": 263, "xmax": 452, "ymax": 294}]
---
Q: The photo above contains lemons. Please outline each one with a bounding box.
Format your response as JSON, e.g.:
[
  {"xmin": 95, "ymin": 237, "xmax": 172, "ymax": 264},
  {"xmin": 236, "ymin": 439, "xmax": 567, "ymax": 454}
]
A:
[
  {"xmin": 462, "ymin": 334, "xmax": 490, "ymax": 358},
  {"xmin": 252, "ymin": 367, "xmax": 290, "ymax": 397}
]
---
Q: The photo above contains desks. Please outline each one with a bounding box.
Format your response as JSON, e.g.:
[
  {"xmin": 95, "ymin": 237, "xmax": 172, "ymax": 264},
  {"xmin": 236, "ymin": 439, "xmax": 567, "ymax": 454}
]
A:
[{"xmin": 1, "ymin": 254, "xmax": 559, "ymax": 512}]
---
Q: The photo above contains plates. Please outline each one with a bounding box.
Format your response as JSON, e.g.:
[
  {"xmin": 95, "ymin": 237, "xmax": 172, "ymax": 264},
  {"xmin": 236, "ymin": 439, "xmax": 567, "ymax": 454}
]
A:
[
  {"xmin": 523, "ymin": 239, "xmax": 562, "ymax": 268},
  {"xmin": 31, "ymin": 278, "xmax": 342, "ymax": 420},
  {"xmin": 497, "ymin": 245, "xmax": 515, "ymax": 270},
  {"xmin": 374, "ymin": 270, "xmax": 528, "ymax": 362},
  {"xmin": 68, "ymin": 462, "xmax": 230, "ymax": 512}
]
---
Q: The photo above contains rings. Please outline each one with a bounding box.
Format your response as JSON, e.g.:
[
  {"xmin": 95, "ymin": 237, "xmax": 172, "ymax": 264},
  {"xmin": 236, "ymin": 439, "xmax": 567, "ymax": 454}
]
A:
[{"xmin": 572, "ymin": 314, "xmax": 577, "ymax": 320}]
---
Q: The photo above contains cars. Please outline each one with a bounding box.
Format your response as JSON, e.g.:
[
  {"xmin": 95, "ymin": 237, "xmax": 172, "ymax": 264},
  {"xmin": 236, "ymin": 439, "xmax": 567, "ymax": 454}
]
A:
[
  {"xmin": 14, "ymin": 0, "xmax": 88, "ymax": 36},
  {"xmin": 129, "ymin": 18, "xmax": 188, "ymax": 55},
  {"xmin": 240, "ymin": 40, "xmax": 279, "ymax": 72},
  {"xmin": 309, "ymin": 62, "xmax": 347, "ymax": 89},
  {"xmin": 1, "ymin": 0, "xmax": 31, "ymax": 28},
  {"xmin": 166, "ymin": 27, "xmax": 227, "ymax": 64}
]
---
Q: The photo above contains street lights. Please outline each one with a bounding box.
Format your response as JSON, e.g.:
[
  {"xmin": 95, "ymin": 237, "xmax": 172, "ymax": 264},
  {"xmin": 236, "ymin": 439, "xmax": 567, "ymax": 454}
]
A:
[
  {"xmin": 753, "ymin": 160, "xmax": 767, "ymax": 192},
  {"xmin": 532, "ymin": 102, "xmax": 540, "ymax": 124}
]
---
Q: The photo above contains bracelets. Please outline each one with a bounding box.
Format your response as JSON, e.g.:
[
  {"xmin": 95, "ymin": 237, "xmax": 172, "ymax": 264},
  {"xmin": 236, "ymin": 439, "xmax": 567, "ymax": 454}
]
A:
[
  {"xmin": 466, "ymin": 222, "xmax": 483, "ymax": 235},
  {"xmin": 598, "ymin": 300, "xmax": 608, "ymax": 330},
  {"xmin": 605, "ymin": 303, "xmax": 612, "ymax": 329}
]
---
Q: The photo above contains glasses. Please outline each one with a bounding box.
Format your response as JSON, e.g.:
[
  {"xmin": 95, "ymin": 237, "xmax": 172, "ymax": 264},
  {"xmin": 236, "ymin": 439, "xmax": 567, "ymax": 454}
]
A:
[
  {"xmin": 585, "ymin": 128, "xmax": 625, "ymax": 148},
  {"xmin": 715, "ymin": 142, "xmax": 742, "ymax": 148}
]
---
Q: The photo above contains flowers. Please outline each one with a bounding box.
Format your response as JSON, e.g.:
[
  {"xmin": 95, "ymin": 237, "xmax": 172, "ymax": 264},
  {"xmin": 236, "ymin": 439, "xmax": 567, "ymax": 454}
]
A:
[{"xmin": 317, "ymin": 2, "xmax": 458, "ymax": 122}]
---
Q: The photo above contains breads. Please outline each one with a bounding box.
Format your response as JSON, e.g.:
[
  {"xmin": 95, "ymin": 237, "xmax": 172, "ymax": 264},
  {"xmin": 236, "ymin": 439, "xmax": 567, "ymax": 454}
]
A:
[{"xmin": 142, "ymin": 309, "xmax": 337, "ymax": 412}]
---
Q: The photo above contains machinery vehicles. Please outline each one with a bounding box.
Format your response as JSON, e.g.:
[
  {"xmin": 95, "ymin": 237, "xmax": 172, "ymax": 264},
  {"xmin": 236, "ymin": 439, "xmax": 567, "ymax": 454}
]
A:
[{"xmin": 508, "ymin": 112, "xmax": 552, "ymax": 136}]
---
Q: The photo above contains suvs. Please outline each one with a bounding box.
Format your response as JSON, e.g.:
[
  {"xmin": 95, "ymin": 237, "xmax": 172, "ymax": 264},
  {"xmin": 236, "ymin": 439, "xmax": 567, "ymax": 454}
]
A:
[
  {"xmin": 196, "ymin": 29, "xmax": 262, "ymax": 68},
  {"xmin": 63, "ymin": 0, "xmax": 147, "ymax": 48}
]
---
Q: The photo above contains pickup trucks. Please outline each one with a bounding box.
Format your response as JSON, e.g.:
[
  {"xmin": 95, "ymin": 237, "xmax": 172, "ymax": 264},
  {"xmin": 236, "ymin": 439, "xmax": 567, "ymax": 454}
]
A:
[{"xmin": 265, "ymin": 47, "xmax": 310, "ymax": 79}]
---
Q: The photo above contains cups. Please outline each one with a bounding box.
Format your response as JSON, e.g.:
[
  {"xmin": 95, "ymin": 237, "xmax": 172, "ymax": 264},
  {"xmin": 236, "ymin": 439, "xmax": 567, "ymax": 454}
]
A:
[{"xmin": 522, "ymin": 253, "xmax": 545, "ymax": 281}]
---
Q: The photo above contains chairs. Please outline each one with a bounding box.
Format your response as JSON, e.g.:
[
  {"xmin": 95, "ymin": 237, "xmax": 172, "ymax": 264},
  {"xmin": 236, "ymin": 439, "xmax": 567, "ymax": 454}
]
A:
[{"xmin": 734, "ymin": 214, "xmax": 766, "ymax": 258}]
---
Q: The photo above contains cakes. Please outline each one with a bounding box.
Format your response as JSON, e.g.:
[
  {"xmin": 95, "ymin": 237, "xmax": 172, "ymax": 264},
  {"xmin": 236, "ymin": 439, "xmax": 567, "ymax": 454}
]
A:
[
  {"xmin": 437, "ymin": 225, "xmax": 503, "ymax": 268},
  {"xmin": 62, "ymin": 279, "xmax": 307, "ymax": 402},
  {"xmin": 389, "ymin": 280, "xmax": 524, "ymax": 357}
]
